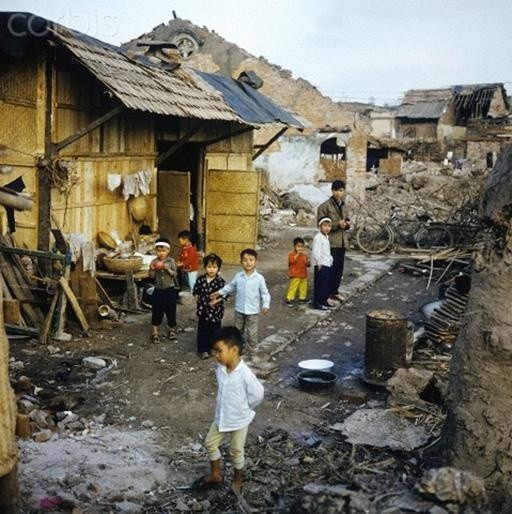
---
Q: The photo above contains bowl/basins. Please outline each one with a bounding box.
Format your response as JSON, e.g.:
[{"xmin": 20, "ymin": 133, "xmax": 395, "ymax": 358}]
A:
[
  {"xmin": 297, "ymin": 370, "xmax": 337, "ymax": 390},
  {"xmin": 298, "ymin": 359, "xmax": 334, "ymax": 373}
]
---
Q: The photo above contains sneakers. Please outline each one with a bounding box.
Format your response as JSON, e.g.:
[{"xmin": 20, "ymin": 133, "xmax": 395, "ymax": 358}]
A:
[
  {"xmin": 299, "ymin": 299, "xmax": 311, "ymax": 303},
  {"xmin": 287, "ymin": 301, "xmax": 293, "ymax": 307}
]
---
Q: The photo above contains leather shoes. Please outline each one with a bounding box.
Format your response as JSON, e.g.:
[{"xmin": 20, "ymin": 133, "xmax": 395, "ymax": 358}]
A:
[
  {"xmin": 314, "ymin": 305, "xmax": 328, "ymax": 310},
  {"xmin": 334, "ymin": 294, "xmax": 344, "ymax": 301},
  {"xmin": 321, "ymin": 303, "xmax": 331, "ymax": 306},
  {"xmin": 327, "ymin": 300, "xmax": 335, "ymax": 305}
]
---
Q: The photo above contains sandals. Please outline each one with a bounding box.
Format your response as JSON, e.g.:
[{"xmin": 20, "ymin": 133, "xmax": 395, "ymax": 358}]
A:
[
  {"xmin": 201, "ymin": 352, "xmax": 209, "ymax": 360},
  {"xmin": 169, "ymin": 331, "xmax": 176, "ymax": 340},
  {"xmin": 152, "ymin": 333, "xmax": 159, "ymax": 344}
]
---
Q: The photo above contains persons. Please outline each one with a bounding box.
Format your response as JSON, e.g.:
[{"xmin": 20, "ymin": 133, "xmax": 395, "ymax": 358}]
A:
[
  {"xmin": 317, "ymin": 180, "xmax": 351, "ymax": 301},
  {"xmin": 311, "ymin": 217, "xmax": 334, "ymax": 310},
  {"xmin": 210, "ymin": 248, "xmax": 271, "ymax": 363},
  {"xmin": 174, "ymin": 230, "xmax": 199, "ymax": 294},
  {"xmin": 193, "ymin": 254, "xmax": 230, "ymax": 359},
  {"xmin": 192, "ymin": 326, "xmax": 264, "ymax": 502},
  {"xmin": 148, "ymin": 238, "xmax": 177, "ymax": 344},
  {"xmin": 286, "ymin": 238, "xmax": 311, "ymax": 306}
]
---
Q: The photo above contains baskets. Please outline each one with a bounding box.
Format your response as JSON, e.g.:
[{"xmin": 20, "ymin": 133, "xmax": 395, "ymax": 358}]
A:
[
  {"xmin": 97, "ymin": 232, "xmax": 117, "ymax": 250},
  {"xmin": 103, "ymin": 255, "xmax": 142, "ymax": 274}
]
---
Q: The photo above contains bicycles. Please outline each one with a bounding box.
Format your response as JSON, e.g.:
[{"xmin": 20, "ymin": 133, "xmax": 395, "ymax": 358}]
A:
[{"xmin": 357, "ymin": 204, "xmax": 452, "ymax": 254}]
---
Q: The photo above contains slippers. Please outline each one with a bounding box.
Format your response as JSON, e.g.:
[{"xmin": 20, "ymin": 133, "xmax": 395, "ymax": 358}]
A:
[
  {"xmin": 193, "ymin": 477, "xmax": 222, "ymax": 487},
  {"xmin": 227, "ymin": 486, "xmax": 243, "ymax": 500}
]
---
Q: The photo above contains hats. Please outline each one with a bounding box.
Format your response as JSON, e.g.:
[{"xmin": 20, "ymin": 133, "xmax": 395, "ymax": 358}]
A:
[
  {"xmin": 318, "ymin": 216, "xmax": 331, "ymax": 225},
  {"xmin": 154, "ymin": 238, "xmax": 170, "ymax": 248}
]
